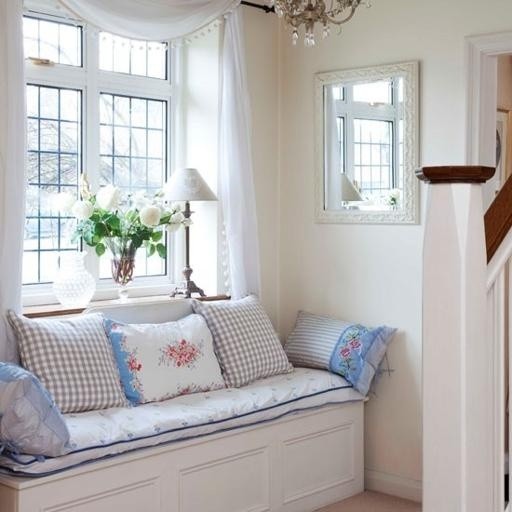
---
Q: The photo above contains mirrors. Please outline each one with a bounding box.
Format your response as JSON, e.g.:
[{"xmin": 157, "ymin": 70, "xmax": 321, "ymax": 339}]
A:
[{"xmin": 313, "ymin": 61, "xmax": 420, "ymax": 226}]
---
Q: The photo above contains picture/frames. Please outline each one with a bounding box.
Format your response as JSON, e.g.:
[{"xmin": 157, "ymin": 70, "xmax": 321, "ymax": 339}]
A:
[{"xmin": 494, "ymin": 108, "xmax": 512, "ymax": 193}]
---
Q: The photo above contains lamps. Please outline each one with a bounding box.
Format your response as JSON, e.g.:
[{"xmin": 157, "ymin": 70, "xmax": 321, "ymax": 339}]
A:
[
  {"xmin": 240, "ymin": 0, "xmax": 373, "ymax": 46},
  {"xmin": 341, "ymin": 174, "xmax": 364, "ymax": 210},
  {"xmin": 154, "ymin": 168, "xmax": 220, "ymax": 297}
]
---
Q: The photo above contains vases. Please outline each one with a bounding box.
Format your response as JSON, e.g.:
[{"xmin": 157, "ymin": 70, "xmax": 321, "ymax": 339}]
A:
[
  {"xmin": 106, "ymin": 237, "xmax": 137, "ymax": 302},
  {"xmin": 51, "ymin": 250, "xmax": 97, "ymax": 309}
]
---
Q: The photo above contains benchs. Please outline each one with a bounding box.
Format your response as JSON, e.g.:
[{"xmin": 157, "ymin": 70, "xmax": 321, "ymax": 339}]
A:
[{"xmin": 0, "ymin": 294, "xmax": 370, "ymax": 512}]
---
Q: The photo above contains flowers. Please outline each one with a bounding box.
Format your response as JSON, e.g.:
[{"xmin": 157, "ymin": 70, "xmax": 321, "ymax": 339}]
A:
[
  {"xmin": 372, "ymin": 188, "xmax": 402, "ymax": 211},
  {"xmin": 53, "ymin": 170, "xmax": 194, "ymax": 284}
]
